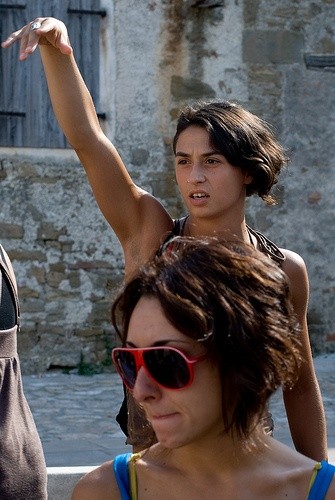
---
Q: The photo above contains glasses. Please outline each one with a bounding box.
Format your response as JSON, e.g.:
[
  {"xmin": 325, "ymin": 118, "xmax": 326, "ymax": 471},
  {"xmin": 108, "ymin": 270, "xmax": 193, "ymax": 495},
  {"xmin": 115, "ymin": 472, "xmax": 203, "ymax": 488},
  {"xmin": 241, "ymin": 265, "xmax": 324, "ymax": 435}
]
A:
[{"xmin": 112, "ymin": 346, "xmax": 211, "ymax": 392}]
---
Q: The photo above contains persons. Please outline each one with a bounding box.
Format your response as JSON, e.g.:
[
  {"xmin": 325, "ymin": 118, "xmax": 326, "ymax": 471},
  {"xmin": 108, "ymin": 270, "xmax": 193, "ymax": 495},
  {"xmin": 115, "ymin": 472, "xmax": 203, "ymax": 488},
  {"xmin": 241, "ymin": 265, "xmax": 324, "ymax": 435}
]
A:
[
  {"xmin": 0, "ymin": 241, "xmax": 48, "ymax": 500},
  {"xmin": 70, "ymin": 231, "xmax": 334, "ymax": 500},
  {"xmin": 1, "ymin": 18, "xmax": 329, "ymax": 463}
]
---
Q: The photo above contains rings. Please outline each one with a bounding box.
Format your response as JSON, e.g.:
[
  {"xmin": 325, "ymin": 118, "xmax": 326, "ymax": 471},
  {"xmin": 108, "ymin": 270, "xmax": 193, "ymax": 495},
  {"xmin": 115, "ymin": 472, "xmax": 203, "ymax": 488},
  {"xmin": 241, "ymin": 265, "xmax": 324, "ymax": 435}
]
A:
[{"xmin": 29, "ymin": 22, "xmax": 41, "ymax": 29}]
